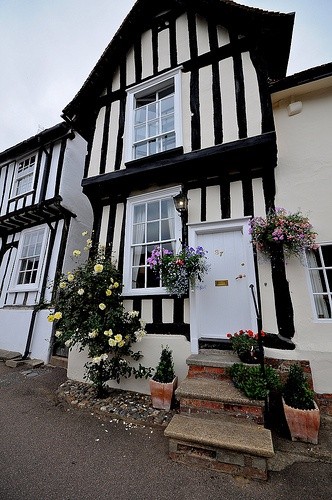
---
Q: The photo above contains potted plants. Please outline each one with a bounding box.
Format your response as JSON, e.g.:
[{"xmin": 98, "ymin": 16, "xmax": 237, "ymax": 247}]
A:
[
  {"xmin": 280, "ymin": 363, "xmax": 320, "ymax": 445},
  {"xmin": 148, "ymin": 344, "xmax": 178, "ymax": 410}
]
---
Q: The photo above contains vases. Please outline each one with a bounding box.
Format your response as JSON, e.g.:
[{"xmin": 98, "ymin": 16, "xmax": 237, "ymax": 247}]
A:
[{"xmin": 235, "ymin": 350, "xmax": 264, "ymax": 365}]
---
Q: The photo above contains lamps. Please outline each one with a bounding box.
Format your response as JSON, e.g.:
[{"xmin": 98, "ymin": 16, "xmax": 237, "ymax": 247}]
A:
[{"xmin": 170, "ymin": 191, "xmax": 189, "ymax": 224}]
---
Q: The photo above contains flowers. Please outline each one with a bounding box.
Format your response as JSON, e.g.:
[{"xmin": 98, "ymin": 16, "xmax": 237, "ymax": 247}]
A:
[
  {"xmin": 146, "ymin": 244, "xmax": 212, "ymax": 299},
  {"xmin": 42, "ymin": 228, "xmax": 154, "ymax": 400},
  {"xmin": 246, "ymin": 207, "xmax": 320, "ymax": 266},
  {"xmin": 226, "ymin": 329, "xmax": 266, "ymax": 357}
]
